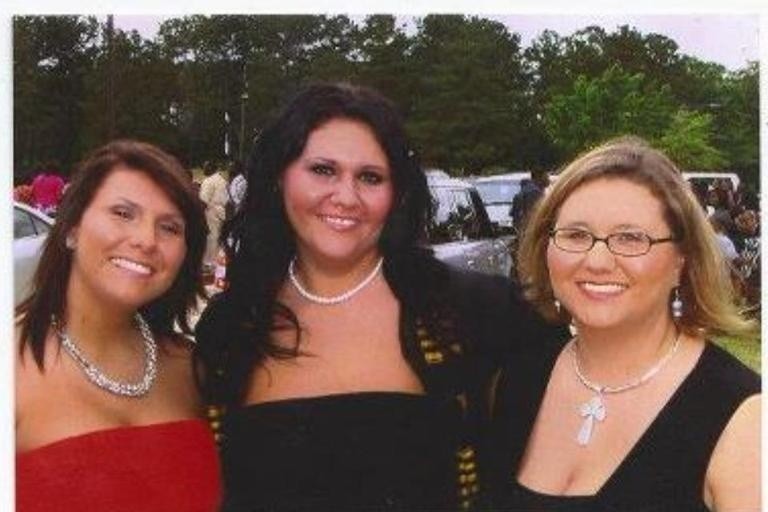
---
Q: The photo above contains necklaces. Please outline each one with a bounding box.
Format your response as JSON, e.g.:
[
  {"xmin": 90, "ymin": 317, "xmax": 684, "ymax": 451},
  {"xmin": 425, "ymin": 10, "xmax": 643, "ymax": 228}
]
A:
[
  {"xmin": 53, "ymin": 308, "xmax": 159, "ymax": 400},
  {"xmin": 568, "ymin": 325, "xmax": 687, "ymax": 447},
  {"xmin": 284, "ymin": 253, "xmax": 385, "ymax": 306}
]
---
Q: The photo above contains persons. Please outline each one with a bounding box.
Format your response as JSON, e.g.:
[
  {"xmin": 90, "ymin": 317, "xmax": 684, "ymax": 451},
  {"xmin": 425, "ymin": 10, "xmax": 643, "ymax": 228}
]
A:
[
  {"xmin": 471, "ymin": 134, "xmax": 763, "ymax": 511},
  {"xmin": 14, "ymin": 159, "xmax": 251, "ymax": 295},
  {"xmin": 190, "ymin": 81, "xmax": 533, "ymax": 510},
  {"xmin": 14, "ymin": 139, "xmax": 230, "ymax": 512},
  {"xmin": 508, "ymin": 162, "xmax": 760, "ymax": 318}
]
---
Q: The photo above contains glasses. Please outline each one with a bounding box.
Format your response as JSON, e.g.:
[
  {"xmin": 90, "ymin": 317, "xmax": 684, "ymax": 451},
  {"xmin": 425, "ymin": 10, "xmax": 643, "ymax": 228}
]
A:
[{"xmin": 546, "ymin": 226, "xmax": 682, "ymax": 258}]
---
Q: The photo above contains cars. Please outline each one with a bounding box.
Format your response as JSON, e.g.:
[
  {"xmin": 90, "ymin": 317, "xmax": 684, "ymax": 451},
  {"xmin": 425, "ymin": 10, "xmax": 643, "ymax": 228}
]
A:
[
  {"xmin": 681, "ymin": 172, "xmax": 741, "ymax": 193},
  {"xmin": 13, "ymin": 199, "xmax": 58, "ymax": 314},
  {"xmin": 417, "ymin": 169, "xmax": 560, "ymax": 283}
]
